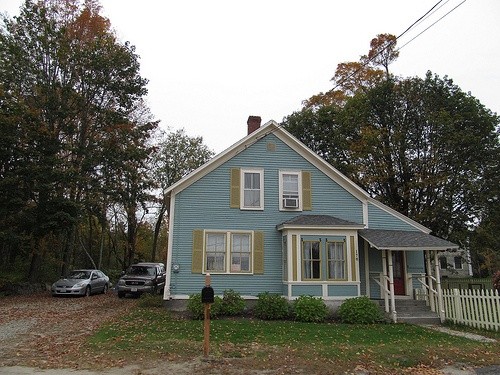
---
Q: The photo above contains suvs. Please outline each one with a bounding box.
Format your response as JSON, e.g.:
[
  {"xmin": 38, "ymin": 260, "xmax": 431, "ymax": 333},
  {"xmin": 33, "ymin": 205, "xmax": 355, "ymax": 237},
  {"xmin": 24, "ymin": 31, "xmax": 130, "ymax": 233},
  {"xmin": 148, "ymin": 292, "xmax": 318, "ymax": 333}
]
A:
[{"xmin": 117, "ymin": 262, "xmax": 166, "ymax": 297}]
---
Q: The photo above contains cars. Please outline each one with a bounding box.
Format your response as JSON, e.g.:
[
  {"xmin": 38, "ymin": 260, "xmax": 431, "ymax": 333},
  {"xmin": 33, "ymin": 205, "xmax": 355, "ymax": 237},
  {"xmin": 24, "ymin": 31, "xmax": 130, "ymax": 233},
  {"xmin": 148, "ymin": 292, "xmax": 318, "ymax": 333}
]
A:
[{"xmin": 51, "ymin": 269, "xmax": 110, "ymax": 297}]
